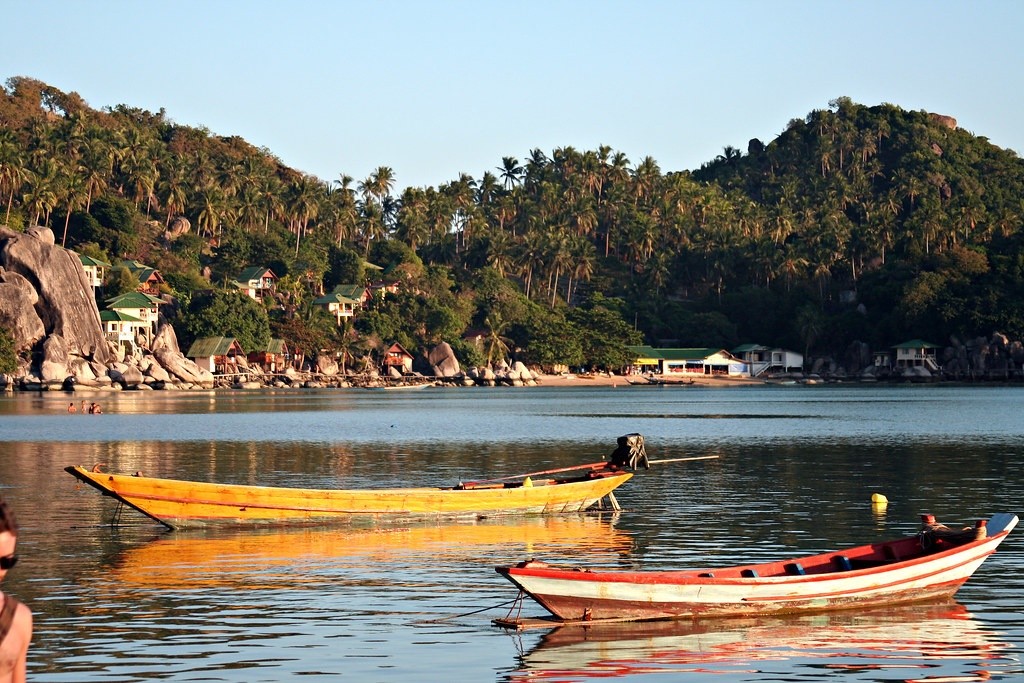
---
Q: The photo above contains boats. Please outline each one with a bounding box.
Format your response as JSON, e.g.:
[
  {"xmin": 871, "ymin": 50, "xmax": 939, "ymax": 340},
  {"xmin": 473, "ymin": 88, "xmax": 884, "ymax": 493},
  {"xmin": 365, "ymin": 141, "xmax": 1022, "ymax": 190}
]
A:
[
  {"xmin": 625, "ymin": 375, "xmax": 696, "ymax": 385},
  {"xmin": 494, "ymin": 595, "xmax": 1015, "ymax": 683},
  {"xmin": 489, "ymin": 511, "xmax": 1020, "ymax": 633},
  {"xmin": 63, "ymin": 432, "xmax": 650, "ymax": 533},
  {"xmin": 93, "ymin": 512, "xmax": 638, "ymax": 586}
]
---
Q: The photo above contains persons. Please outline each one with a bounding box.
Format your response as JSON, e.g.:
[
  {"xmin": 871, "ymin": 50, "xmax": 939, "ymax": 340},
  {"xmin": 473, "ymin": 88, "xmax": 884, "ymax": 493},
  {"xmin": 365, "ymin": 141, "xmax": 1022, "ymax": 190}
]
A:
[
  {"xmin": 69, "ymin": 400, "xmax": 103, "ymax": 414},
  {"xmin": 0, "ymin": 498, "xmax": 34, "ymax": 683}
]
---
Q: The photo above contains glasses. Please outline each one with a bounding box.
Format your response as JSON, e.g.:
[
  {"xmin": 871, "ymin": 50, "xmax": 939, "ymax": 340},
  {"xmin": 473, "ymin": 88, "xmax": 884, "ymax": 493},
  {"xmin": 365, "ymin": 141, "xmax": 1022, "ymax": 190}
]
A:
[{"xmin": 0, "ymin": 554, "xmax": 18, "ymax": 569}]
